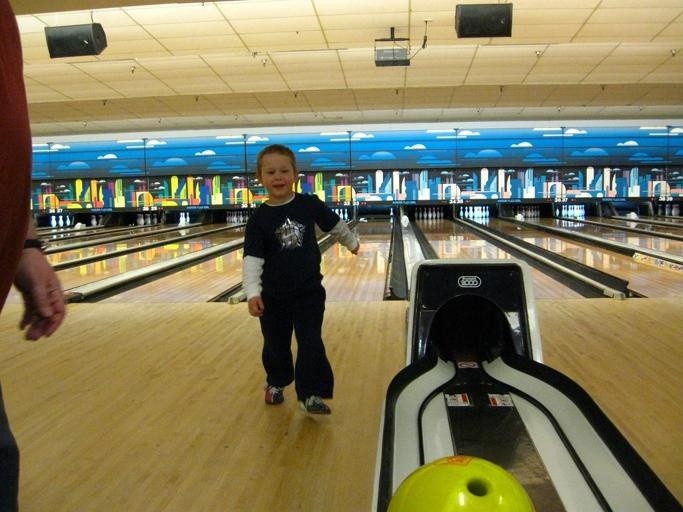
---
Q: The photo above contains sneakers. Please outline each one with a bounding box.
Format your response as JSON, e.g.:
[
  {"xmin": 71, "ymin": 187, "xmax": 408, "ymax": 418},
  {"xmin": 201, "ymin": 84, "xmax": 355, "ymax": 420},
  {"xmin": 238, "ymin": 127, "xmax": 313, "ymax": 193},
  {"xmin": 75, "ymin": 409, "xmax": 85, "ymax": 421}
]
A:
[
  {"xmin": 263, "ymin": 383, "xmax": 285, "ymax": 405},
  {"xmin": 298, "ymin": 393, "xmax": 332, "ymax": 415}
]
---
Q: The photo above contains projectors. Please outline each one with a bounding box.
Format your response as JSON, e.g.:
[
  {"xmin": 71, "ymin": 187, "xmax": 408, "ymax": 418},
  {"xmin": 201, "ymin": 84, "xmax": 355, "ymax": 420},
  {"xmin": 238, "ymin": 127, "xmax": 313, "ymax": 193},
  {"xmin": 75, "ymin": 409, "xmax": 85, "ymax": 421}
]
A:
[{"xmin": 377, "ymin": 48, "xmax": 407, "ymax": 62}]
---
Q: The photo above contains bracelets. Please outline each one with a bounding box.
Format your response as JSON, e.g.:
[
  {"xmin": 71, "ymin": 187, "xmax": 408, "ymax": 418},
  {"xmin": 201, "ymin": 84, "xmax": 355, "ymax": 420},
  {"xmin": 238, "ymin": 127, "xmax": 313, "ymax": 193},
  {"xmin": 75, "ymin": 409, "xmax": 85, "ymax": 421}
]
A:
[{"xmin": 23, "ymin": 239, "xmax": 41, "ymax": 249}]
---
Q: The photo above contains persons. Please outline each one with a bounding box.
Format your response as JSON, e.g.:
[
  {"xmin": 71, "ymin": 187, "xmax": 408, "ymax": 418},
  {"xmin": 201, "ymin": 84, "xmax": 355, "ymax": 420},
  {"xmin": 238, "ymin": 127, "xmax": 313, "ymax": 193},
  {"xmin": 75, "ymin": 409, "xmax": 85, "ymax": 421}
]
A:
[
  {"xmin": 0, "ymin": 1, "xmax": 71, "ymax": 510},
  {"xmin": 241, "ymin": 143, "xmax": 360, "ymax": 414}
]
